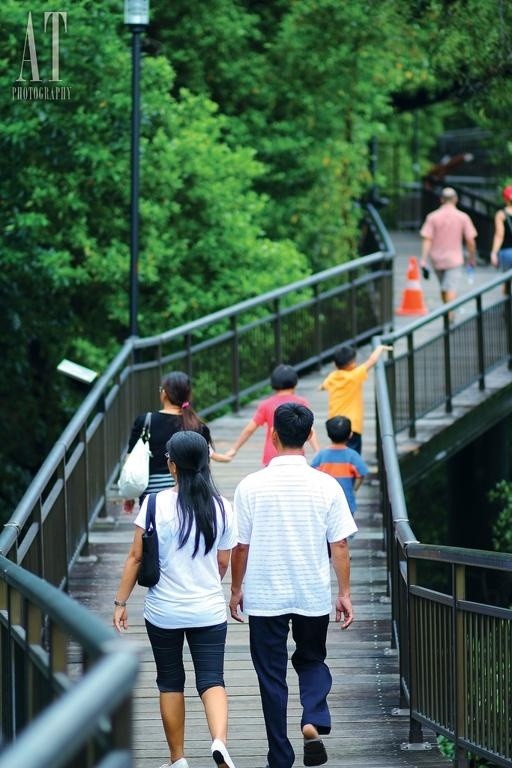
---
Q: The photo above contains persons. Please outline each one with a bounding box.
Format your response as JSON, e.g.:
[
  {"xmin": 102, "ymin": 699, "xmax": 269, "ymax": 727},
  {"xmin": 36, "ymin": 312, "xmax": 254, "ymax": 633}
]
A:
[
  {"xmin": 491, "ymin": 186, "xmax": 512, "ymax": 291},
  {"xmin": 421, "ymin": 187, "xmax": 477, "ymax": 322},
  {"xmin": 230, "ymin": 364, "xmax": 322, "ymax": 464},
  {"xmin": 317, "ymin": 342, "xmax": 395, "ymax": 458},
  {"xmin": 111, "ymin": 432, "xmax": 240, "ymax": 768},
  {"xmin": 230, "ymin": 404, "xmax": 356, "ymax": 767},
  {"xmin": 120, "ymin": 371, "xmax": 228, "ymax": 515},
  {"xmin": 313, "ymin": 417, "xmax": 367, "ymax": 514}
]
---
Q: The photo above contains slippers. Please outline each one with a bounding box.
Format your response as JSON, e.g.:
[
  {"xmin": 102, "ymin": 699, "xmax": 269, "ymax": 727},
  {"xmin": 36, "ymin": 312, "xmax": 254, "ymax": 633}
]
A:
[{"xmin": 303, "ymin": 739, "xmax": 327, "ymax": 766}]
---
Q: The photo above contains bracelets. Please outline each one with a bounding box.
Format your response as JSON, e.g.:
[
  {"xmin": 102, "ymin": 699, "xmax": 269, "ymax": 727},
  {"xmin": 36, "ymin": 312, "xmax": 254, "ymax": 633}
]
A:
[{"xmin": 112, "ymin": 600, "xmax": 127, "ymax": 610}]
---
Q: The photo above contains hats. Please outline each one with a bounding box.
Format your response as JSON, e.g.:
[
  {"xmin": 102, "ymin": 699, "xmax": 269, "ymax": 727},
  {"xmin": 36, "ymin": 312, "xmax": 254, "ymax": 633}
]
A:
[{"xmin": 504, "ymin": 187, "xmax": 512, "ymax": 200}]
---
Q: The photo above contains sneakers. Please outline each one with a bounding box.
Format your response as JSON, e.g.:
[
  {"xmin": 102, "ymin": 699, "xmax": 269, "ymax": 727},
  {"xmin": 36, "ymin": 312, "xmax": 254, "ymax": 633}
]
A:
[
  {"xmin": 212, "ymin": 740, "xmax": 236, "ymax": 768},
  {"xmin": 160, "ymin": 758, "xmax": 188, "ymax": 768}
]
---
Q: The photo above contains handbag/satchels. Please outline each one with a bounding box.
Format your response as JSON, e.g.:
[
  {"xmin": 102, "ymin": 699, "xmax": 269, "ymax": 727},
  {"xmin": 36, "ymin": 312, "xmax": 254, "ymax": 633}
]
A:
[
  {"xmin": 138, "ymin": 530, "xmax": 160, "ymax": 587},
  {"xmin": 117, "ymin": 439, "xmax": 151, "ymax": 499}
]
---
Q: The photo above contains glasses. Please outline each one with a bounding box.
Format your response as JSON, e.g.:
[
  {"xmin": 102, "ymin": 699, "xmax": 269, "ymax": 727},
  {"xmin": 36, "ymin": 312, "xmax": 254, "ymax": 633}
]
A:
[{"xmin": 165, "ymin": 453, "xmax": 169, "ymax": 458}]
